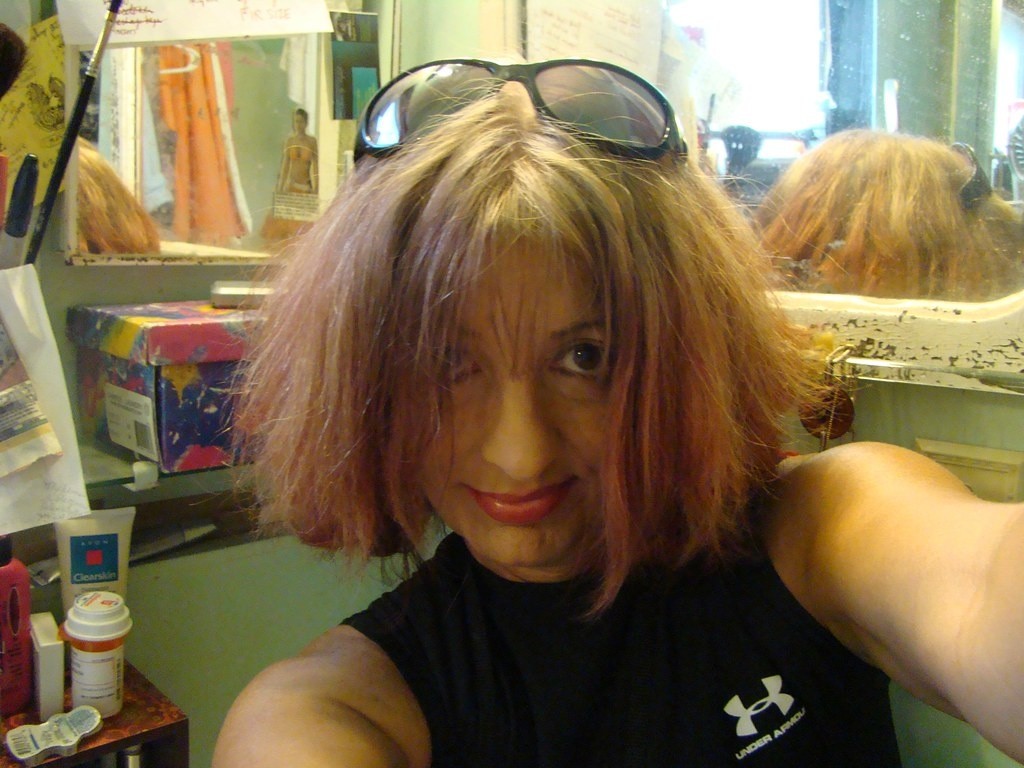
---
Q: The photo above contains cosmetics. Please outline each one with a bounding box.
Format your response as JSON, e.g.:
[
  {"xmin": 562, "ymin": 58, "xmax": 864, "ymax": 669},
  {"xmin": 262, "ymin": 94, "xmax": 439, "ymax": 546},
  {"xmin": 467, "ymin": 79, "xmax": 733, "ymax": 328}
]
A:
[{"xmin": 0, "ymin": 506, "xmax": 136, "ymax": 768}]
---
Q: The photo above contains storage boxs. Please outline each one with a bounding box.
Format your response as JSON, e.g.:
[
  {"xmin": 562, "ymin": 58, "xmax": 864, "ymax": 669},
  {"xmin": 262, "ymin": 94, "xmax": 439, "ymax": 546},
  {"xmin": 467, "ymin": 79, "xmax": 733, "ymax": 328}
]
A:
[
  {"xmin": 65, "ymin": 299, "xmax": 265, "ymax": 476},
  {"xmin": 29, "ymin": 611, "xmax": 65, "ymax": 723}
]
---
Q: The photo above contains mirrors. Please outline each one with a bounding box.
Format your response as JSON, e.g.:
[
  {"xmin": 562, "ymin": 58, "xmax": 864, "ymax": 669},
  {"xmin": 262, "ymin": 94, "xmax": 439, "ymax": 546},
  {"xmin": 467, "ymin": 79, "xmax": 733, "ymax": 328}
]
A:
[
  {"xmin": 66, "ymin": 29, "xmax": 334, "ymax": 267},
  {"xmin": 393, "ymin": 0, "xmax": 1024, "ymax": 399}
]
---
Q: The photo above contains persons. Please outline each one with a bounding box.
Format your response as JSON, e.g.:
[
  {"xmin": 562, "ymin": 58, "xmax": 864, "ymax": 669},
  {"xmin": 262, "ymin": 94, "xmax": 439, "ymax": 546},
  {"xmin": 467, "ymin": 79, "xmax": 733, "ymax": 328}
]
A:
[
  {"xmin": 77, "ymin": 135, "xmax": 160, "ymax": 255},
  {"xmin": 214, "ymin": 80, "xmax": 1024, "ymax": 768},
  {"xmin": 748, "ymin": 127, "xmax": 1024, "ymax": 304},
  {"xmin": 277, "ymin": 108, "xmax": 318, "ymax": 194}
]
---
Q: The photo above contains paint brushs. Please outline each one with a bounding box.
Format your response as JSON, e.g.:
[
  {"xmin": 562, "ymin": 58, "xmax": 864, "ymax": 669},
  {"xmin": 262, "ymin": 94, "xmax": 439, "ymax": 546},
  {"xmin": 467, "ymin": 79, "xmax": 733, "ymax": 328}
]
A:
[{"xmin": 22, "ymin": 0, "xmax": 127, "ymax": 264}]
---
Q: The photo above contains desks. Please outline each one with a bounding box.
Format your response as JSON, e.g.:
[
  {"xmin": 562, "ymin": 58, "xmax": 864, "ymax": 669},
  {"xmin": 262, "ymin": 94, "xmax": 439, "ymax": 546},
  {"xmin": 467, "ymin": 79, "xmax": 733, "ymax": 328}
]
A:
[{"xmin": 0, "ymin": 644, "xmax": 190, "ymax": 768}]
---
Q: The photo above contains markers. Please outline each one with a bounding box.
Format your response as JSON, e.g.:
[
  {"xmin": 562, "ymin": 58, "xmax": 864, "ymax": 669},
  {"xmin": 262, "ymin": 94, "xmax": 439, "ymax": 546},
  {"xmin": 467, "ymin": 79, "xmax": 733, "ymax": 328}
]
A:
[{"xmin": 2, "ymin": 153, "xmax": 40, "ymax": 268}]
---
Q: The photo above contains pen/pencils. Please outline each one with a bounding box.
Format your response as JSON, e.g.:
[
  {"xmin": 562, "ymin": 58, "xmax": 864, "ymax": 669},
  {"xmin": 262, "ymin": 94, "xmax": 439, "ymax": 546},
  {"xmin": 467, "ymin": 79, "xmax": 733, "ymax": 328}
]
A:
[{"xmin": 0, "ymin": 154, "xmax": 8, "ymax": 233}]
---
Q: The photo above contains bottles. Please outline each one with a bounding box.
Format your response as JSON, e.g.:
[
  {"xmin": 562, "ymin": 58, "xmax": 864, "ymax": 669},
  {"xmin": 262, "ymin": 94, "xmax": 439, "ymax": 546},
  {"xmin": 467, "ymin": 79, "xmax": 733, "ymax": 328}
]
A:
[{"xmin": 63, "ymin": 591, "xmax": 133, "ymax": 719}]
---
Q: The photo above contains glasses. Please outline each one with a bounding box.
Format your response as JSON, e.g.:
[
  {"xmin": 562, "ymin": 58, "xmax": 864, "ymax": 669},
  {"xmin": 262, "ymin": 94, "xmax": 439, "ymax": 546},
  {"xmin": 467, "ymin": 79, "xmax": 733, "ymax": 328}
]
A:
[
  {"xmin": 949, "ymin": 142, "xmax": 992, "ymax": 214},
  {"xmin": 353, "ymin": 58, "xmax": 689, "ymax": 167}
]
